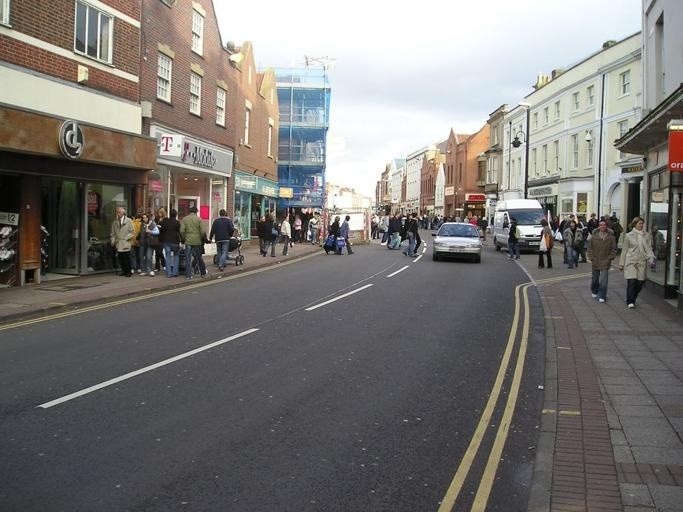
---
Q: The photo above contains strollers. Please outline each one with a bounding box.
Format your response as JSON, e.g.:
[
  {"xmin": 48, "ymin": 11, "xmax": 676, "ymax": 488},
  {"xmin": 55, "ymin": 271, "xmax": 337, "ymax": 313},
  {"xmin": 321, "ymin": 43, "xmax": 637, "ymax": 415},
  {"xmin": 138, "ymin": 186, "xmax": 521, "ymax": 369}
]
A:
[
  {"xmin": 213, "ymin": 227, "xmax": 243, "ymax": 266},
  {"xmin": 173, "ymin": 240, "xmax": 199, "ymax": 276}
]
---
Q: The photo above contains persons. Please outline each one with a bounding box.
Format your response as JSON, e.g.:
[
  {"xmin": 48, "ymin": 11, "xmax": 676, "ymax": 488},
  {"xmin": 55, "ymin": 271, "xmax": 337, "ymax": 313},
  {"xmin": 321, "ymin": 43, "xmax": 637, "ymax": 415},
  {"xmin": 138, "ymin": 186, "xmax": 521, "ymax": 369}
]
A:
[
  {"xmin": 588, "ymin": 220, "xmax": 618, "ymax": 303},
  {"xmin": 109, "ymin": 205, "xmax": 235, "ymax": 279},
  {"xmin": 507, "ymin": 218, "xmax": 520, "ymax": 260},
  {"xmin": 620, "ymin": 217, "xmax": 656, "ymax": 310},
  {"xmin": 372, "ymin": 211, "xmax": 422, "ymax": 257},
  {"xmin": 326, "ymin": 216, "xmax": 354, "ymax": 255},
  {"xmin": 418, "ymin": 214, "xmax": 488, "ymax": 242},
  {"xmin": 258, "ymin": 212, "xmax": 320, "ymax": 258},
  {"xmin": 551, "ymin": 213, "xmax": 622, "ymax": 268},
  {"xmin": 652, "ymin": 223, "xmax": 665, "ymax": 272},
  {"xmin": 537, "ymin": 219, "xmax": 552, "ymax": 269}
]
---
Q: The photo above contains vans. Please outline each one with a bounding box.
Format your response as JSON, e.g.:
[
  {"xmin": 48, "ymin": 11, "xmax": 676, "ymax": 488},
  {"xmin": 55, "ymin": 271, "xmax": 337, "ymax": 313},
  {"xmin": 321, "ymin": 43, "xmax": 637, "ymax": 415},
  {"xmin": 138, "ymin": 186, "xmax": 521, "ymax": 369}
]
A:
[
  {"xmin": 650, "ymin": 202, "xmax": 670, "ymax": 244},
  {"xmin": 492, "ymin": 199, "xmax": 555, "ymax": 253}
]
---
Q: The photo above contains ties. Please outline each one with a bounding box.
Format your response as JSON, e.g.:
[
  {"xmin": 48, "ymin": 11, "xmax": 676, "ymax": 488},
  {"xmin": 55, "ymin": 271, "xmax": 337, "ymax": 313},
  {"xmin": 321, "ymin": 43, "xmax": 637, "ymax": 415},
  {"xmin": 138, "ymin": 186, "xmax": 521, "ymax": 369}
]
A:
[{"xmin": 119, "ymin": 219, "xmax": 121, "ymax": 229}]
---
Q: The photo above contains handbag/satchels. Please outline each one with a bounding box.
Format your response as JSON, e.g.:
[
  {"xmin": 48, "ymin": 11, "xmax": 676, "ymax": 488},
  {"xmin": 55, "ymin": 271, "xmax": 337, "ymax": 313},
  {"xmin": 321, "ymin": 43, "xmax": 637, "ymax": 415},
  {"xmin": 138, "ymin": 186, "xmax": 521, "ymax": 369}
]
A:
[
  {"xmin": 145, "ymin": 236, "xmax": 161, "ymax": 248},
  {"xmin": 271, "ymin": 227, "xmax": 279, "ymax": 236}
]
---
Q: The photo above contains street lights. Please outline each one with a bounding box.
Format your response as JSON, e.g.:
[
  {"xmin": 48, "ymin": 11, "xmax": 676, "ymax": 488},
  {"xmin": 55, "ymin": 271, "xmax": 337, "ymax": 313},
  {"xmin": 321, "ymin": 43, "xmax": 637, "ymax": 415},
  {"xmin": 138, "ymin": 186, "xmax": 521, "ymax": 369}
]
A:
[{"xmin": 510, "ymin": 107, "xmax": 531, "ymax": 200}]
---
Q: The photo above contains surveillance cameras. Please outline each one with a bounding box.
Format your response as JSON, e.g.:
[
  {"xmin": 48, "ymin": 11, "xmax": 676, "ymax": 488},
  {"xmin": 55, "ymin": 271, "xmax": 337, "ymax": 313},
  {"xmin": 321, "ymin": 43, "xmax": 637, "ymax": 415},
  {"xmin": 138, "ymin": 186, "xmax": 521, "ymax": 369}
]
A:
[
  {"xmin": 642, "ymin": 155, "xmax": 649, "ymax": 161},
  {"xmin": 584, "ymin": 128, "xmax": 593, "ymax": 142}
]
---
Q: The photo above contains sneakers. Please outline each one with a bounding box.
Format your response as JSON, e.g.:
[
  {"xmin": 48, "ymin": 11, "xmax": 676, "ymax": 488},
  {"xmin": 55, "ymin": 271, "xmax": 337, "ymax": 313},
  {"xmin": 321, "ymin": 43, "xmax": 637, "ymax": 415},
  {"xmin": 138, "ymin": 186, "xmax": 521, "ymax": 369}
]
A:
[
  {"xmin": 599, "ymin": 298, "xmax": 605, "ymax": 303},
  {"xmin": 627, "ymin": 303, "xmax": 635, "ymax": 309},
  {"xmin": 130, "ymin": 266, "xmax": 167, "ymax": 277},
  {"xmin": 591, "ymin": 293, "xmax": 597, "ymax": 298}
]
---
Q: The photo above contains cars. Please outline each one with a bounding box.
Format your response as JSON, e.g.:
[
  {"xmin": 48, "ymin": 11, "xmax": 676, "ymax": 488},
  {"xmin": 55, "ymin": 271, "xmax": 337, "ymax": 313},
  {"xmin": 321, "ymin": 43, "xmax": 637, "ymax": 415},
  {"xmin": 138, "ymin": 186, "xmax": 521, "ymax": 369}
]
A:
[
  {"xmin": 249, "ymin": 220, "xmax": 261, "ymax": 236},
  {"xmin": 431, "ymin": 221, "xmax": 484, "ymax": 263},
  {"xmin": 376, "ymin": 216, "xmax": 407, "ymax": 233}
]
